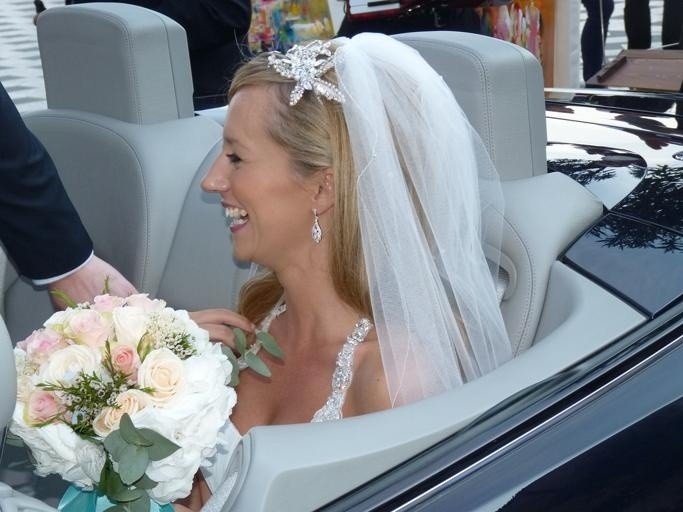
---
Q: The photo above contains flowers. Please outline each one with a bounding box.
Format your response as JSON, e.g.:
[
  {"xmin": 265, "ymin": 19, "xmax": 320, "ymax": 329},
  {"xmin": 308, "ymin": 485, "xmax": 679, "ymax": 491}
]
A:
[{"xmin": 10, "ymin": 291, "xmax": 239, "ymax": 511}]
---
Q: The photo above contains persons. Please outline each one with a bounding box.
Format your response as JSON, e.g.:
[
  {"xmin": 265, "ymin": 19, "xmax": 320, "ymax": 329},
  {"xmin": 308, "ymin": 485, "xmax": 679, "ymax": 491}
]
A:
[
  {"xmin": 582, "ymin": 0, "xmax": 614, "ymax": 79},
  {"xmin": 163, "ymin": 32, "xmax": 515, "ymax": 512},
  {"xmin": 0, "ymin": 79, "xmax": 139, "ymax": 320},
  {"xmin": 624, "ymin": 0, "xmax": 683, "ymax": 51}
]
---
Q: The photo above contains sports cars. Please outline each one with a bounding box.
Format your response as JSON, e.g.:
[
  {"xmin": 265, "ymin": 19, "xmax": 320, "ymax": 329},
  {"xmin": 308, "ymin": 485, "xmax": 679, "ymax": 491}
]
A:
[{"xmin": 0, "ymin": 2, "xmax": 683, "ymax": 512}]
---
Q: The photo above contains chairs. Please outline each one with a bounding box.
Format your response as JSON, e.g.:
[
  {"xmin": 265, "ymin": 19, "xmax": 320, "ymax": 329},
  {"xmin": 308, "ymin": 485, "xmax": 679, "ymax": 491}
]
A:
[
  {"xmin": 155, "ymin": 31, "xmax": 572, "ymax": 361},
  {"xmin": 0, "ymin": 4, "xmax": 224, "ymax": 432}
]
